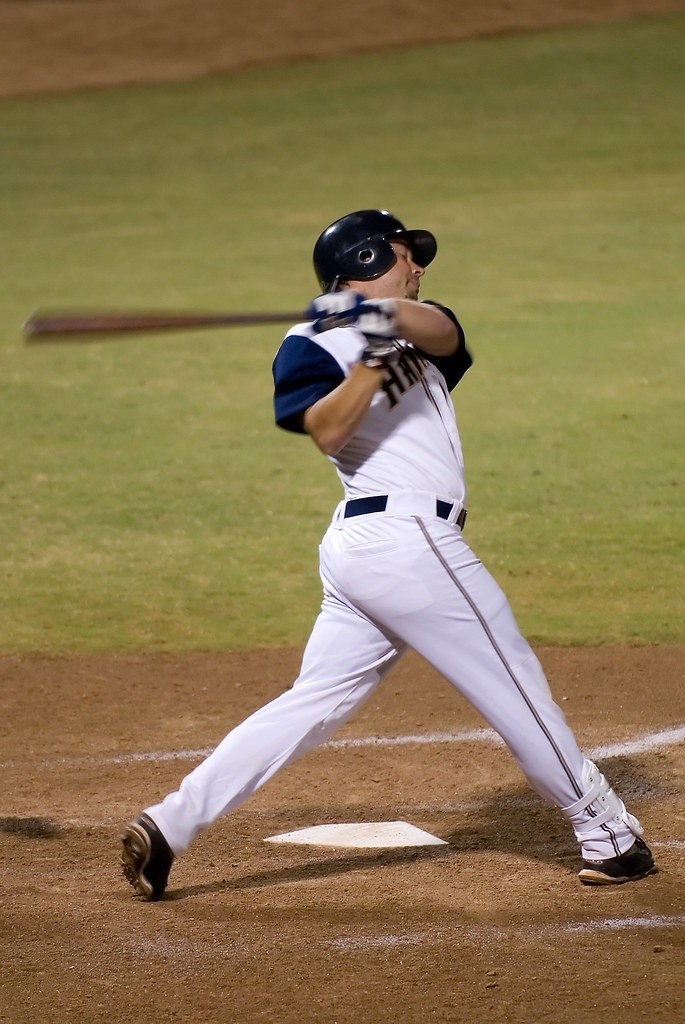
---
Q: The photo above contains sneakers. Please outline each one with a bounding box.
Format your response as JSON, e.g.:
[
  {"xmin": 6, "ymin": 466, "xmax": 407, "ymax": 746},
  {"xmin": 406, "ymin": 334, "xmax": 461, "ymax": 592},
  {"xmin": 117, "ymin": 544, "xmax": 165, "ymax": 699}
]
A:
[
  {"xmin": 578, "ymin": 836, "xmax": 655, "ymax": 884},
  {"xmin": 119, "ymin": 814, "xmax": 174, "ymax": 902}
]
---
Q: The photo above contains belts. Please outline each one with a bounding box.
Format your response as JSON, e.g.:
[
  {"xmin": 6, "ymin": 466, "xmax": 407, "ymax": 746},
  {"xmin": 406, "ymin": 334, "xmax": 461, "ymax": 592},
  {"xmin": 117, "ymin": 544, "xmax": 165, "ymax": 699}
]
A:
[{"xmin": 338, "ymin": 496, "xmax": 467, "ymax": 532}]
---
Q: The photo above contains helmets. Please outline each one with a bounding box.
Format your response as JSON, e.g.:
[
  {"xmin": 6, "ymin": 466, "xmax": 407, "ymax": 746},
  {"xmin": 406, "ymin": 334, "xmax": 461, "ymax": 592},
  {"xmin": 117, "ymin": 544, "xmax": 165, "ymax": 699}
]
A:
[{"xmin": 313, "ymin": 209, "xmax": 437, "ymax": 294}]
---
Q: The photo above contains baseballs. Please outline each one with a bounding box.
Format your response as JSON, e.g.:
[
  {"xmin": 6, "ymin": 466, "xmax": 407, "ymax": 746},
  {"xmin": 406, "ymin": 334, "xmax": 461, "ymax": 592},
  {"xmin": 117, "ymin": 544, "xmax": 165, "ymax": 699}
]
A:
[{"xmin": 22, "ymin": 305, "xmax": 320, "ymax": 346}]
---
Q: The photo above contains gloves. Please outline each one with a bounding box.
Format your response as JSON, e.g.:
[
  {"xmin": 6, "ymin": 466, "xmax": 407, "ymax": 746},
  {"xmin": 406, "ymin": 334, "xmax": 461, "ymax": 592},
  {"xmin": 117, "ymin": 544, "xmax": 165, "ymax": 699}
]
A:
[
  {"xmin": 304, "ymin": 291, "xmax": 367, "ymax": 328},
  {"xmin": 357, "ymin": 297, "xmax": 407, "ymax": 367}
]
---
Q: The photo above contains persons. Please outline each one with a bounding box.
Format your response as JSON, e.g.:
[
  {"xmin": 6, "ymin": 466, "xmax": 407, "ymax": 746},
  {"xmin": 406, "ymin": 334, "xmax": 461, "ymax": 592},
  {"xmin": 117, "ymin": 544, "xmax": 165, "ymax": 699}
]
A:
[{"xmin": 118, "ymin": 209, "xmax": 658, "ymax": 901}]
